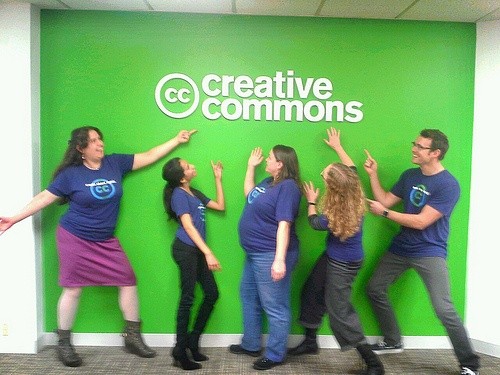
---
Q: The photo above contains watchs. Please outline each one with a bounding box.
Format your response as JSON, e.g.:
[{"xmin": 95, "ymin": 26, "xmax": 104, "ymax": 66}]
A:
[{"xmin": 383, "ymin": 208, "xmax": 389, "ymax": 217}]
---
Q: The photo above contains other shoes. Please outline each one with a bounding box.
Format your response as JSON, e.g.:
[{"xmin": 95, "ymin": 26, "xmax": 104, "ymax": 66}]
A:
[
  {"xmin": 230, "ymin": 345, "xmax": 261, "ymax": 357},
  {"xmin": 366, "ymin": 363, "xmax": 386, "ymax": 375},
  {"xmin": 287, "ymin": 340, "xmax": 318, "ymax": 356},
  {"xmin": 253, "ymin": 356, "xmax": 282, "ymax": 370}
]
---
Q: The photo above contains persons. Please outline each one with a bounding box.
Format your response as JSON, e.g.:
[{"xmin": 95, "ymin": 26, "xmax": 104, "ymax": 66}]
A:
[
  {"xmin": 229, "ymin": 145, "xmax": 302, "ymax": 370},
  {"xmin": 0, "ymin": 125, "xmax": 197, "ymax": 366},
  {"xmin": 162, "ymin": 158, "xmax": 227, "ymax": 370},
  {"xmin": 363, "ymin": 129, "xmax": 481, "ymax": 375},
  {"xmin": 286, "ymin": 127, "xmax": 386, "ymax": 375}
]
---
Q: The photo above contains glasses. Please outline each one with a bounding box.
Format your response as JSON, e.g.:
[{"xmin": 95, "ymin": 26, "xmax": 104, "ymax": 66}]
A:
[{"xmin": 412, "ymin": 142, "xmax": 439, "ymax": 149}]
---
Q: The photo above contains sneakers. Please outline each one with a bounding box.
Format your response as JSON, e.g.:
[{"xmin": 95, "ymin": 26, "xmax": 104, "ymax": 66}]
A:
[
  {"xmin": 369, "ymin": 338, "xmax": 404, "ymax": 355},
  {"xmin": 459, "ymin": 367, "xmax": 480, "ymax": 375}
]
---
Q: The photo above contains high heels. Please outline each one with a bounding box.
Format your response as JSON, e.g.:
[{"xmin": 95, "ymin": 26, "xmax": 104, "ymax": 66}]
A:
[
  {"xmin": 172, "ymin": 345, "xmax": 202, "ymax": 370},
  {"xmin": 186, "ymin": 342, "xmax": 207, "ymax": 361}
]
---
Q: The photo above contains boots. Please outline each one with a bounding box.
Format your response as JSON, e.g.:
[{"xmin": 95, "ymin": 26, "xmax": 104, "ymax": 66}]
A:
[
  {"xmin": 124, "ymin": 319, "xmax": 156, "ymax": 358},
  {"xmin": 55, "ymin": 328, "xmax": 82, "ymax": 367}
]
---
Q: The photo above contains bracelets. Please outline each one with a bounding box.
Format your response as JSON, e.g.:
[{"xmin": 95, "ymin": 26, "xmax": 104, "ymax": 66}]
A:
[{"xmin": 308, "ymin": 202, "xmax": 316, "ymax": 205}]
